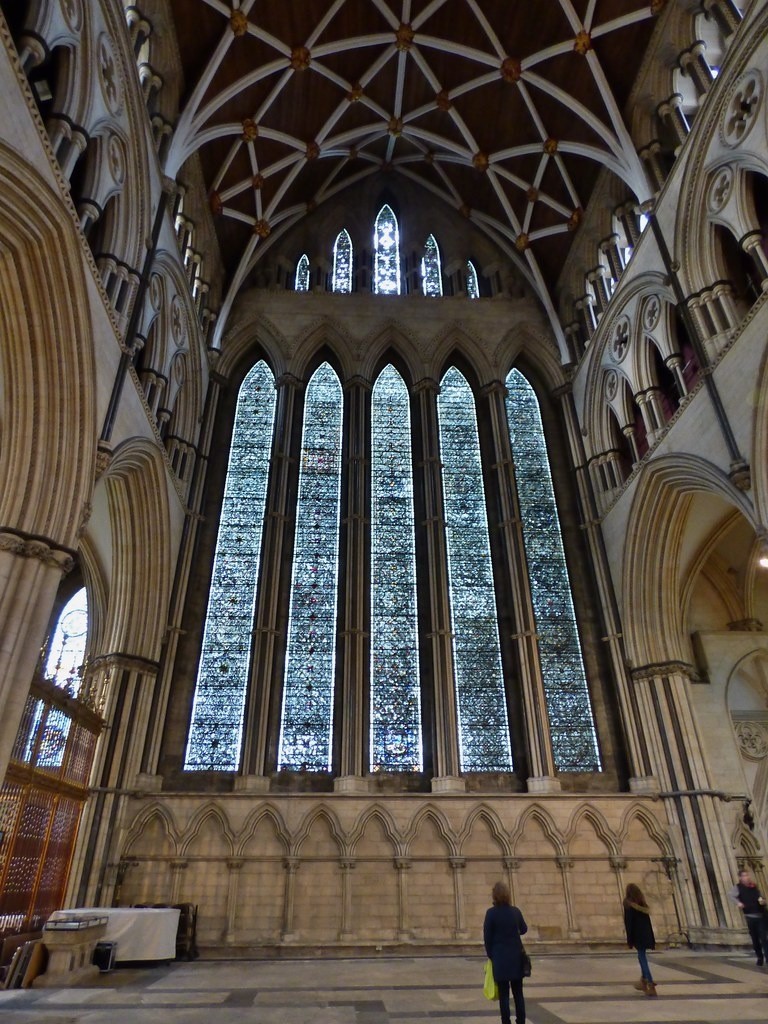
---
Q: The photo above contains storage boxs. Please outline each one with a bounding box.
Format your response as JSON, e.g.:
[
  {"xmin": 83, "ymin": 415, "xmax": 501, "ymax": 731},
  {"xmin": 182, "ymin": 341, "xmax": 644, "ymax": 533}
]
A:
[{"xmin": 93, "ymin": 941, "xmax": 118, "ymax": 971}]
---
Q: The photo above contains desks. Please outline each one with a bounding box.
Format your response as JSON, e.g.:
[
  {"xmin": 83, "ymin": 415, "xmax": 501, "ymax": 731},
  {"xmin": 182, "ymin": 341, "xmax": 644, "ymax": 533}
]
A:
[{"xmin": 48, "ymin": 908, "xmax": 181, "ymax": 966}]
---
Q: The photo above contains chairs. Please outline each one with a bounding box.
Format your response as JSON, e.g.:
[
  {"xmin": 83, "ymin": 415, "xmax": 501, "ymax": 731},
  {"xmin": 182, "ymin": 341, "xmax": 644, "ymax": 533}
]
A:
[{"xmin": 115, "ymin": 902, "xmax": 199, "ymax": 963}]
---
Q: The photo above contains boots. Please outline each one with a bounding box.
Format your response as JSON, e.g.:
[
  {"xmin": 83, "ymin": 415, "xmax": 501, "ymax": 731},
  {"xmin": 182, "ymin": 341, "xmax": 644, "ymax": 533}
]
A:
[
  {"xmin": 634, "ymin": 976, "xmax": 648, "ymax": 990},
  {"xmin": 645, "ymin": 983, "xmax": 658, "ymax": 997}
]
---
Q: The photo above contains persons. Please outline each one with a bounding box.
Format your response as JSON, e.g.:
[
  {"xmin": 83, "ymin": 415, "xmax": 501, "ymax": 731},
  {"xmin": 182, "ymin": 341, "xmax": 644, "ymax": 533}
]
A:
[
  {"xmin": 623, "ymin": 883, "xmax": 658, "ymax": 997},
  {"xmin": 483, "ymin": 882, "xmax": 528, "ymax": 1024},
  {"xmin": 727, "ymin": 870, "xmax": 768, "ymax": 965}
]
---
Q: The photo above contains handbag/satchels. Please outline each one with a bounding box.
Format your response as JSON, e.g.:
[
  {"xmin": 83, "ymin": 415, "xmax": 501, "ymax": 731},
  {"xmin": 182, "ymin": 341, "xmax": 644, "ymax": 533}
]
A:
[
  {"xmin": 523, "ymin": 955, "xmax": 532, "ymax": 977},
  {"xmin": 483, "ymin": 959, "xmax": 499, "ymax": 1002}
]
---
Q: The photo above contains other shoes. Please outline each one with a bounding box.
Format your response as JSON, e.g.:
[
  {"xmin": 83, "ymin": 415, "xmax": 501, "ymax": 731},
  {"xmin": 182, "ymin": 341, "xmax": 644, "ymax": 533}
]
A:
[
  {"xmin": 755, "ymin": 958, "xmax": 764, "ymax": 966},
  {"xmin": 764, "ymin": 957, "xmax": 768, "ymax": 964}
]
